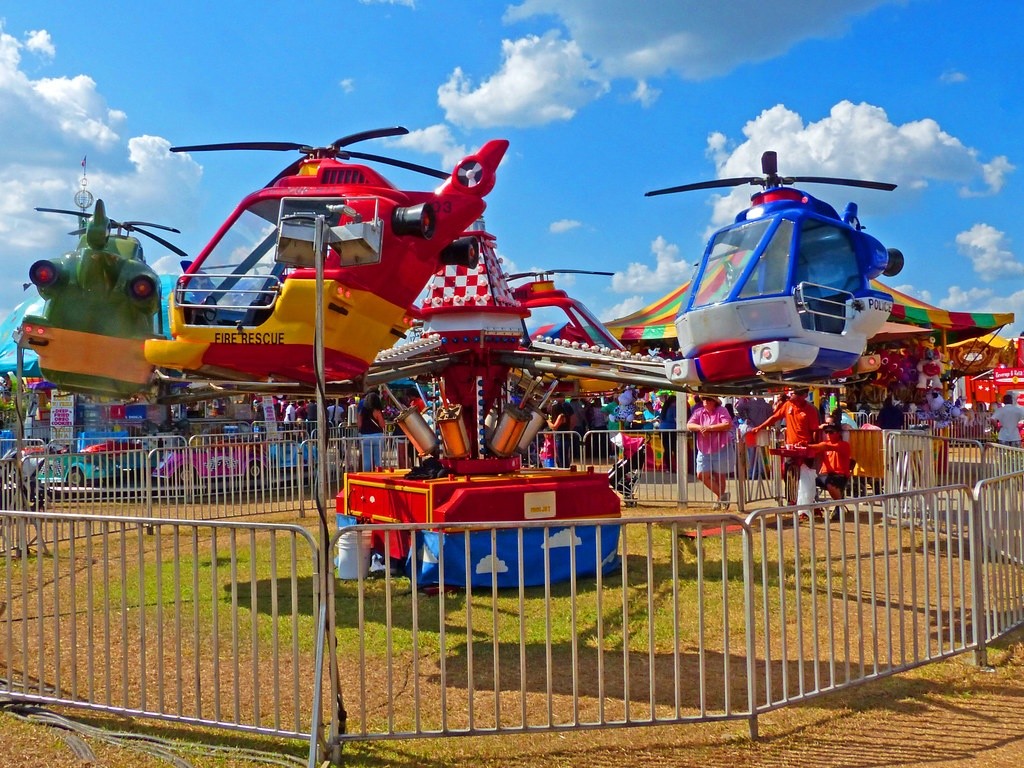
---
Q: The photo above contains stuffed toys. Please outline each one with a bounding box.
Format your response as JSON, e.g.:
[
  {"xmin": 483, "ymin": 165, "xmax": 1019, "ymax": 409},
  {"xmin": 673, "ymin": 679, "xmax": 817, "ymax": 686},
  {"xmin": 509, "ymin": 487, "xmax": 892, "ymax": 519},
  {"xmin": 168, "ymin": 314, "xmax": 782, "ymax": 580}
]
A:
[
  {"xmin": 862, "ymin": 344, "xmax": 919, "ymax": 404},
  {"xmin": 609, "ymin": 388, "xmax": 636, "ymax": 423},
  {"xmin": 914, "ymin": 336, "xmax": 966, "ymax": 429}
]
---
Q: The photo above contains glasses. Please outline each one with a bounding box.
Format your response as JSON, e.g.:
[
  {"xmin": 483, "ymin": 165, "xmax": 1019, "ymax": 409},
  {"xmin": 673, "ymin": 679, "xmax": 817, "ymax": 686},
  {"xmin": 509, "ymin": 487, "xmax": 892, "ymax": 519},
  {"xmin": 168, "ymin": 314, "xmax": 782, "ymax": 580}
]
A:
[
  {"xmin": 790, "ymin": 392, "xmax": 798, "ymax": 396},
  {"xmin": 824, "ymin": 429, "xmax": 840, "ymax": 434}
]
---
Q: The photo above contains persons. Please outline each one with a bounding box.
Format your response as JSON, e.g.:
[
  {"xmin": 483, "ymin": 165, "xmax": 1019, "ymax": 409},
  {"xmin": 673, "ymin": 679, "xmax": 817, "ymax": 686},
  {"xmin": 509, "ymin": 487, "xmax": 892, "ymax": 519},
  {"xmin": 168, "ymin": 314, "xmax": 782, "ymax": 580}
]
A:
[
  {"xmin": 186, "ymin": 364, "xmax": 905, "ymax": 521},
  {"xmin": 990, "ymin": 394, "xmax": 1024, "ymax": 448},
  {"xmin": 2, "ymin": 374, "xmax": 13, "ymax": 396}
]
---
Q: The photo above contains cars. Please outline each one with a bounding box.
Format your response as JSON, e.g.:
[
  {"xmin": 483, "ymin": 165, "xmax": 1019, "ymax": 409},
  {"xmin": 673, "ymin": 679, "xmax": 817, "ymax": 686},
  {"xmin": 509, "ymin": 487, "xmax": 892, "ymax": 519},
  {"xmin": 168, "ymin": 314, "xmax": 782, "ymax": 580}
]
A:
[{"xmin": 1, "ymin": 439, "xmax": 319, "ymax": 488}]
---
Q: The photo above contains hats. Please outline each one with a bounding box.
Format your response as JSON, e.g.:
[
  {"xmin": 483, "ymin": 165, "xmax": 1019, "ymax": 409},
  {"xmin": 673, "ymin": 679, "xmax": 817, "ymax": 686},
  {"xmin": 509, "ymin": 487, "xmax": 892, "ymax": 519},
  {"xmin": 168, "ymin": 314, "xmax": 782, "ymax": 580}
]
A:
[
  {"xmin": 699, "ymin": 394, "xmax": 722, "ymax": 405},
  {"xmin": 823, "ymin": 425, "xmax": 841, "ymax": 430},
  {"xmin": 289, "ymin": 401, "xmax": 295, "ymax": 404}
]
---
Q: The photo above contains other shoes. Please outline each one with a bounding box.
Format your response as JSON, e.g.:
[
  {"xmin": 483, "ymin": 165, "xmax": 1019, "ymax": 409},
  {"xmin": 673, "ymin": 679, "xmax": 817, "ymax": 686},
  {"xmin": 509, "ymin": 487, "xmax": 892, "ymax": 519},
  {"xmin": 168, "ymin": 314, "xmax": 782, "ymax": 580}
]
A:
[
  {"xmin": 830, "ymin": 507, "xmax": 840, "ymax": 520},
  {"xmin": 722, "ymin": 493, "xmax": 730, "ymax": 510},
  {"xmin": 798, "ymin": 513, "xmax": 809, "ymax": 523},
  {"xmin": 712, "ymin": 497, "xmax": 722, "ymax": 510}
]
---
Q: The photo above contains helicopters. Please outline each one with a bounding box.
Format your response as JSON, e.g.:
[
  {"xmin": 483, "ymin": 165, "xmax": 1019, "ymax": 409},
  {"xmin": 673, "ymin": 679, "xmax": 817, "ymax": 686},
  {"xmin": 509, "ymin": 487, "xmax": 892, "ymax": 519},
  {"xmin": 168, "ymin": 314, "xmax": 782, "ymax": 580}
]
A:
[
  {"xmin": 143, "ymin": 126, "xmax": 510, "ymax": 391},
  {"xmin": 642, "ymin": 153, "xmax": 904, "ymax": 390},
  {"xmin": 17, "ymin": 197, "xmax": 191, "ymax": 399},
  {"xmin": 496, "ymin": 265, "xmax": 631, "ymax": 395}
]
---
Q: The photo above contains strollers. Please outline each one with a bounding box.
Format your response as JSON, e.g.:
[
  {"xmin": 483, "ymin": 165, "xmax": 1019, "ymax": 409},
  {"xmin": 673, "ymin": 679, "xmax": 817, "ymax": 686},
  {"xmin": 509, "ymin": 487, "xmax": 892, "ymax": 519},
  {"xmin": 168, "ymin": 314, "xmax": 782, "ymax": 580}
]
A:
[{"xmin": 605, "ymin": 432, "xmax": 652, "ymax": 507}]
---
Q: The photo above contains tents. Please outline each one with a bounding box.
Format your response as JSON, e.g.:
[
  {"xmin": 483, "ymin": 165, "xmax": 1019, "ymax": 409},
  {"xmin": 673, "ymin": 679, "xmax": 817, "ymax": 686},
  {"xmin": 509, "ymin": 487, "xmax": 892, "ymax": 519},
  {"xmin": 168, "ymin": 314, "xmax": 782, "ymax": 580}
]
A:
[{"xmin": 566, "ymin": 241, "xmax": 1016, "ymax": 437}]
